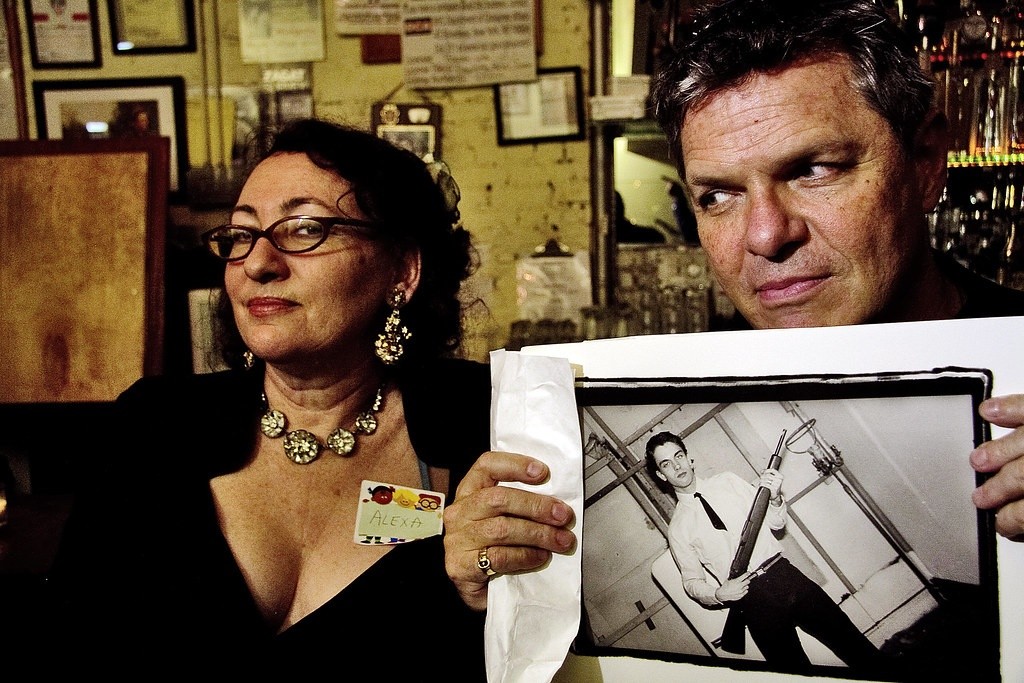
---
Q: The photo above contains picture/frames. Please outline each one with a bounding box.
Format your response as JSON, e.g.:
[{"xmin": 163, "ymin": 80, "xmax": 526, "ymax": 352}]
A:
[
  {"xmin": 31, "ymin": 75, "xmax": 190, "ymax": 186},
  {"xmin": 491, "ymin": 65, "xmax": 587, "ymax": 145},
  {"xmin": 22, "ymin": 0, "xmax": 104, "ymax": 71},
  {"xmin": 107, "ymin": 0, "xmax": 197, "ymax": 58}
]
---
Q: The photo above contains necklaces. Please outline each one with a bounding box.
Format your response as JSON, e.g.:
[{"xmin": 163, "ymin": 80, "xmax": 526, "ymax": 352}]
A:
[{"xmin": 256, "ymin": 383, "xmax": 391, "ymax": 466}]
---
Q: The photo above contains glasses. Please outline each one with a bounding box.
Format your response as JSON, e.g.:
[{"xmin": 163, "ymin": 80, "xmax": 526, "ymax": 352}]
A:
[{"xmin": 199, "ymin": 215, "xmax": 410, "ymax": 262}]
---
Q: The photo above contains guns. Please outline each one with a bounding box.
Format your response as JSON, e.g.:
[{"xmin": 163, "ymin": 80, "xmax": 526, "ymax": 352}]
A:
[{"xmin": 709, "ymin": 421, "xmax": 788, "ymax": 657}]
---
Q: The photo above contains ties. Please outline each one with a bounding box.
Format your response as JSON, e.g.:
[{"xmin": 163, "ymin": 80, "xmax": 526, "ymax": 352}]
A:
[{"xmin": 694, "ymin": 492, "xmax": 727, "ymax": 530}]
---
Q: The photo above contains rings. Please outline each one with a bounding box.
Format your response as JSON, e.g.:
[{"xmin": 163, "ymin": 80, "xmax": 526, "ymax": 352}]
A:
[{"xmin": 478, "ymin": 547, "xmax": 496, "ymax": 577}]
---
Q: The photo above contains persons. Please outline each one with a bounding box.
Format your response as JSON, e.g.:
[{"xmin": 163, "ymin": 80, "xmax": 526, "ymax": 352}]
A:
[
  {"xmin": 646, "ymin": 428, "xmax": 907, "ymax": 683},
  {"xmin": 445, "ymin": 0, "xmax": 1024, "ymax": 683},
  {"xmin": 0, "ymin": 118, "xmax": 491, "ymax": 683}
]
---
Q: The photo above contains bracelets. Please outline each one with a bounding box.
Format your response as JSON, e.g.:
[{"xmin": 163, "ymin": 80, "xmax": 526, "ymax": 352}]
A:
[{"xmin": 769, "ymin": 495, "xmax": 779, "ymax": 501}]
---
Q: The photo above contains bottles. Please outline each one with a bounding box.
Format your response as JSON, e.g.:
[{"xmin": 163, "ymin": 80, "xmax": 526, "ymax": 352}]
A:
[{"xmin": 877, "ymin": 0, "xmax": 1024, "ymax": 288}]
